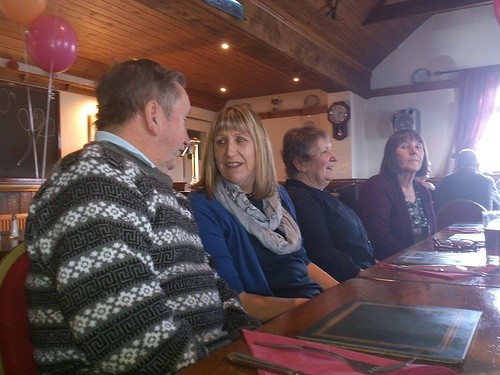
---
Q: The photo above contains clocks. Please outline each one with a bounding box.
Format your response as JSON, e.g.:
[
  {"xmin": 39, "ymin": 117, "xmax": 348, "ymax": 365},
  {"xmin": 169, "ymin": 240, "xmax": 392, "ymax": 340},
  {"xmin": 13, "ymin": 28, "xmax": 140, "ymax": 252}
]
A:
[{"xmin": 327, "ymin": 102, "xmax": 351, "ymax": 141}]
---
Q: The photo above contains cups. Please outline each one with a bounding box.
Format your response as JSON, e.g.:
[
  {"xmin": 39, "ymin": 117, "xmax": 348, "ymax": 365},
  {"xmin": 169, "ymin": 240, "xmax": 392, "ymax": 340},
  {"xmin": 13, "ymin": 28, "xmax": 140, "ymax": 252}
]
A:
[{"xmin": 481, "ymin": 210, "xmax": 500, "ymax": 262}]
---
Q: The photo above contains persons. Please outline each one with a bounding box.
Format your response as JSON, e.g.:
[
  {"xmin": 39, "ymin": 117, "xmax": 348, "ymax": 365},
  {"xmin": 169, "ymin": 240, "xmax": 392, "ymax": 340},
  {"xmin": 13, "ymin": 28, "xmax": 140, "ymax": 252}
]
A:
[
  {"xmin": 361, "ymin": 128, "xmax": 438, "ymax": 260},
  {"xmin": 184, "ymin": 101, "xmax": 343, "ymax": 323},
  {"xmin": 25, "ymin": 59, "xmax": 261, "ymax": 375},
  {"xmin": 437, "ymin": 149, "xmax": 499, "ymax": 213},
  {"xmin": 278, "ymin": 126, "xmax": 381, "ymax": 281}
]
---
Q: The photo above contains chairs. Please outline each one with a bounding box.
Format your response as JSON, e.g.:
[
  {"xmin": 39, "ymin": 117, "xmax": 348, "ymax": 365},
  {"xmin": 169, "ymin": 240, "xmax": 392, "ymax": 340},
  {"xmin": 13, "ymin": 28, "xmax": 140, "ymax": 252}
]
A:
[{"xmin": 0, "ymin": 242, "xmax": 40, "ymax": 375}]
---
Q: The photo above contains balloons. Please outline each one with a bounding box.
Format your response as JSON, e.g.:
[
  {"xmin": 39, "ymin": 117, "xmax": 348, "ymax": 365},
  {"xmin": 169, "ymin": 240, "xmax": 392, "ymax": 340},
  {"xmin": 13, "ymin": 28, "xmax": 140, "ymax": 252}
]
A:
[
  {"xmin": 0, "ymin": 0, "xmax": 48, "ymax": 24},
  {"xmin": 27, "ymin": 14, "xmax": 78, "ymax": 73}
]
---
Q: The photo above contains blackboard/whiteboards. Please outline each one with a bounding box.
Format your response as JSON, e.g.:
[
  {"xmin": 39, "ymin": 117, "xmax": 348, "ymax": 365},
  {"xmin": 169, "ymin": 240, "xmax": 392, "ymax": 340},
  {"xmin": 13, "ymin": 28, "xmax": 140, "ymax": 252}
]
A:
[{"xmin": 0, "ymin": 82, "xmax": 59, "ymax": 179}]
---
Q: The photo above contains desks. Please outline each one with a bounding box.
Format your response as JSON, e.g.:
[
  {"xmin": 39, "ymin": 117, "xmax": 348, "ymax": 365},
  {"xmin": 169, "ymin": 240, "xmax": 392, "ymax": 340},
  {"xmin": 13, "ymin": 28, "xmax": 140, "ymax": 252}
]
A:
[
  {"xmin": 0, "ymin": 230, "xmax": 25, "ymax": 256},
  {"xmin": 174, "ymin": 222, "xmax": 500, "ymax": 375}
]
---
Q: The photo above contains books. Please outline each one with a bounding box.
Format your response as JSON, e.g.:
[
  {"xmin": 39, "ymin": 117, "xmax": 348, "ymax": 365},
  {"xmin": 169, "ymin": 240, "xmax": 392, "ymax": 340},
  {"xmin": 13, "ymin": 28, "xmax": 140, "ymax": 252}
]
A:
[
  {"xmin": 297, "ymin": 299, "xmax": 482, "ymax": 362},
  {"xmin": 393, "ymin": 250, "xmax": 486, "ymax": 268}
]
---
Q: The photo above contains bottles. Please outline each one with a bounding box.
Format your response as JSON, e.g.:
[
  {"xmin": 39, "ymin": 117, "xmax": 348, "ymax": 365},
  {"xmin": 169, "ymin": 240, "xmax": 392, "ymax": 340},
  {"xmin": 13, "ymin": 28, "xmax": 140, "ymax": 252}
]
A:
[{"xmin": 11, "ymin": 214, "xmax": 19, "ymax": 238}]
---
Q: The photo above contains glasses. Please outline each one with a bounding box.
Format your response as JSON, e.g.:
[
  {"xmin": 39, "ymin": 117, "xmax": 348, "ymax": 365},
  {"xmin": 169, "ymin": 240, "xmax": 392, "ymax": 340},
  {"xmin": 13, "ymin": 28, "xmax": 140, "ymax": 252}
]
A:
[{"xmin": 433, "ymin": 237, "xmax": 477, "ymax": 252}]
